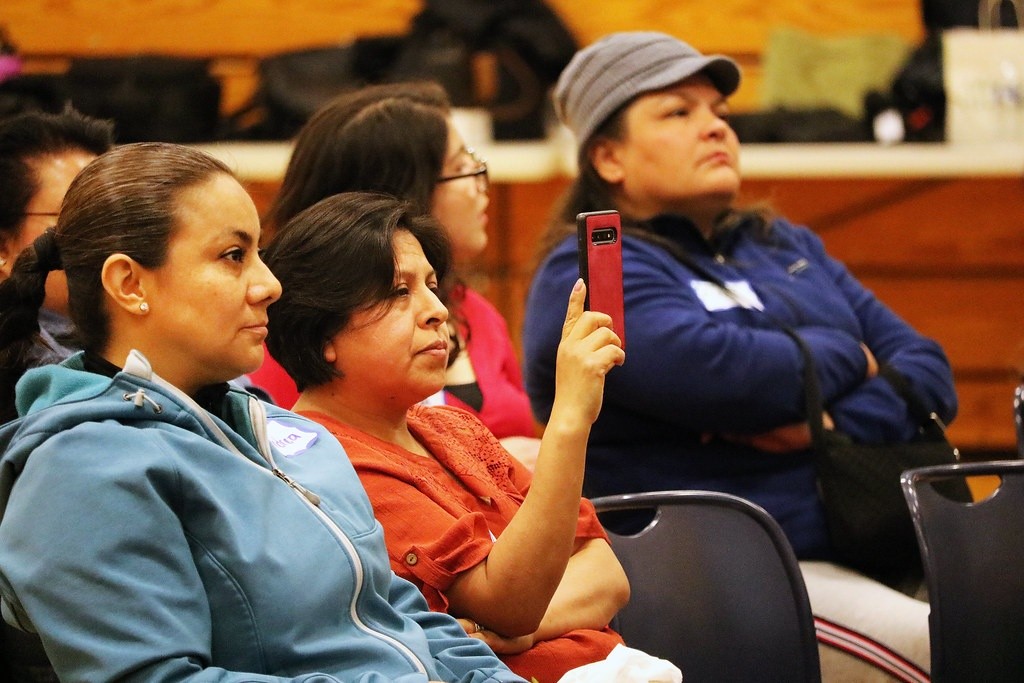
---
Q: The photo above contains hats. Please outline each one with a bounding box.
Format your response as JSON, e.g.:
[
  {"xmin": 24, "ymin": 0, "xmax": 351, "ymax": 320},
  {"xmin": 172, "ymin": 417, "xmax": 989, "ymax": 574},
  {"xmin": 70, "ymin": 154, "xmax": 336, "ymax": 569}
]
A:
[{"xmin": 552, "ymin": 32, "xmax": 742, "ymax": 146}]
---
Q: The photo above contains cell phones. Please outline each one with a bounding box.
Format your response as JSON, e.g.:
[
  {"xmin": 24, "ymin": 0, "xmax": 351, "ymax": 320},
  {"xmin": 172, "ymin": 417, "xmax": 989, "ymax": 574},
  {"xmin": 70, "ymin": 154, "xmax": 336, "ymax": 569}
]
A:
[{"xmin": 576, "ymin": 210, "xmax": 626, "ymax": 352}]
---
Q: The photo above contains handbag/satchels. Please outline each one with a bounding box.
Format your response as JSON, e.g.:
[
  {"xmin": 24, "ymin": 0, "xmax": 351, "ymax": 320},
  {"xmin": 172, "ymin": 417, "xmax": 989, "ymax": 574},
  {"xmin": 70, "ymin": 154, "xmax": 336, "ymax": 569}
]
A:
[{"xmin": 780, "ymin": 322, "xmax": 974, "ymax": 590}]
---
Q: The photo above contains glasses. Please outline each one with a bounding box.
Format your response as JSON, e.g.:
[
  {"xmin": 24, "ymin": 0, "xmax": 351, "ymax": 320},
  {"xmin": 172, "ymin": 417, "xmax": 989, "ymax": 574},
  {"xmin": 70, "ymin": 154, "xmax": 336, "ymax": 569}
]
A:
[{"xmin": 434, "ymin": 146, "xmax": 491, "ymax": 195}]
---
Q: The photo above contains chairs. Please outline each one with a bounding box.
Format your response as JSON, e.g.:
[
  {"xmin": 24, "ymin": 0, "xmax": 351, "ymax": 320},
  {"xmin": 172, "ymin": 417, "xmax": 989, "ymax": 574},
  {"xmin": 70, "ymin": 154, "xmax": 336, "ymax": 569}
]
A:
[
  {"xmin": 899, "ymin": 461, "xmax": 1024, "ymax": 683},
  {"xmin": 588, "ymin": 489, "xmax": 822, "ymax": 683}
]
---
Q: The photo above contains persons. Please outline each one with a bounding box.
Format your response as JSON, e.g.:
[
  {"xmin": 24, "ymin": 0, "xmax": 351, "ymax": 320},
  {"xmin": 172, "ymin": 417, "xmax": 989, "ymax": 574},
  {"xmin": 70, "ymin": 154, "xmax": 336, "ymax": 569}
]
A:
[
  {"xmin": 0, "ymin": 142, "xmax": 530, "ymax": 683},
  {"xmin": 0, "ymin": 106, "xmax": 113, "ymax": 365},
  {"xmin": 253, "ymin": 192, "xmax": 683, "ymax": 683},
  {"xmin": 523, "ymin": 29, "xmax": 958, "ymax": 683},
  {"xmin": 276, "ymin": 78, "xmax": 546, "ymax": 475}
]
